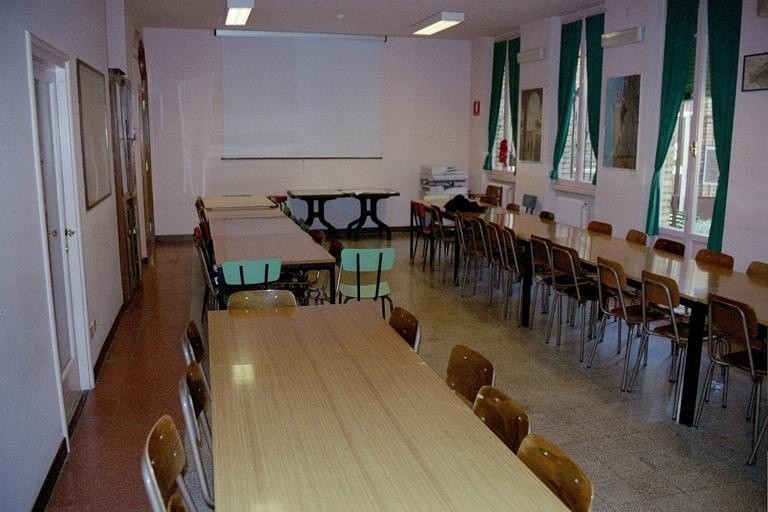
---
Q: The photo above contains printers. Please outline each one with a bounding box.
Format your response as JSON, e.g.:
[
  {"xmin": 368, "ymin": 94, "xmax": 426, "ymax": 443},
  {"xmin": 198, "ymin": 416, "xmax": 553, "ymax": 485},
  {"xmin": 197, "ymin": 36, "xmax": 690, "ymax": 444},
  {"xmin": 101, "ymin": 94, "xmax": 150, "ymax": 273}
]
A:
[{"xmin": 420, "ymin": 162, "xmax": 466, "ymax": 182}]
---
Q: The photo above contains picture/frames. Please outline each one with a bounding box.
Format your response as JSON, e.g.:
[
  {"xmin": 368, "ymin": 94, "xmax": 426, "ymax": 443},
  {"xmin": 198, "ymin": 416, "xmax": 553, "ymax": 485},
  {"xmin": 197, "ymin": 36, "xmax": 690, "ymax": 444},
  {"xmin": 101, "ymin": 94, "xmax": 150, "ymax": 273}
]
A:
[
  {"xmin": 741, "ymin": 53, "xmax": 768, "ymax": 93},
  {"xmin": 76, "ymin": 59, "xmax": 112, "ymax": 210}
]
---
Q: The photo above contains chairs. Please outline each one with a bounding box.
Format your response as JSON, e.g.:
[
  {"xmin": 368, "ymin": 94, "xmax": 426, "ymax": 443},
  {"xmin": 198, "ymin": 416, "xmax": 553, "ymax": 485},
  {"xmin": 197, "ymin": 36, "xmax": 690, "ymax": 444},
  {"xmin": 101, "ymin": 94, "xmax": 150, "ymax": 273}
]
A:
[
  {"xmin": 446, "ymin": 345, "xmax": 496, "ymax": 410},
  {"xmin": 389, "ymin": 307, "xmax": 421, "ymax": 355},
  {"xmin": 193, "ymin": 196, "xmax": 218, "ymax": 321},
  {"xmin": 178, "ymin": 362, "xmax": 214, "ymax": 509},
  {"xmin": 182, "ymin": 318, "xmax": 210, "ymax": 400},
  {"xmin": 472, "ymin": 386, "xmax": 530, "ymax": 455},
  {"xmin": 140, "ymin": 415, "xmax": 200, "ymax": 512},
  {"xmin": 339, "ymin": 248, "xmax": 394, "ymax": 320},
  {"xmin": 516, "ymin": 434, "xmax": 593, "ymax": 511}
]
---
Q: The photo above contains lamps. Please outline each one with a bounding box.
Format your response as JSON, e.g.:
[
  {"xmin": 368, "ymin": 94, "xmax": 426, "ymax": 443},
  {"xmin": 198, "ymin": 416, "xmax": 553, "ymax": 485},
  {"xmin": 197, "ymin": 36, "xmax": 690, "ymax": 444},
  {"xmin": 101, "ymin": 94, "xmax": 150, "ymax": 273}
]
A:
[
  {"xmin": 226, "ymin": 0, "xmax": 254, "ymax": 26},
  {"xmin": 409, "ymin": 12, "xmax": 465, "ymax": 35}
]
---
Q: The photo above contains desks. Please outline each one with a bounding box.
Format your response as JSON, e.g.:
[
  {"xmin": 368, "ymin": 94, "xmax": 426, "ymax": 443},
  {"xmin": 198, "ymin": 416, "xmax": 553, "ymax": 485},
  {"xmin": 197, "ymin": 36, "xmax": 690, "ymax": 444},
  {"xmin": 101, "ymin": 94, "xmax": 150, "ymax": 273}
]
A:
[
  {"xmin": 203, "ymin": 187, "xmax": 401, "ymax": 310},
  {"xmin": 208, "ymin": 301, "xmax": 573, "ymax": 511}
]
